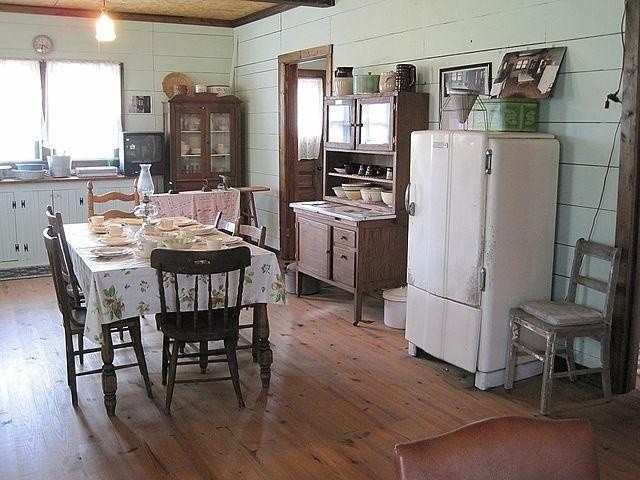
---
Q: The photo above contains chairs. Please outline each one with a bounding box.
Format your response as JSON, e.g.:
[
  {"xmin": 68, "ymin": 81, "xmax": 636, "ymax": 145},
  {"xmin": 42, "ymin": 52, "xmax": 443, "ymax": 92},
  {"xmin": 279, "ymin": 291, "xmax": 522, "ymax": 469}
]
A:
[
  {"xmin": 84, "ymin": 178, "xmax": 141, "ymax": 222},
  {"xmin": 150, "ymin": 246, "xmax": 251, "ymax": 413},
  {"xmin": 42, "ymin": 224, "xmax": 154, "ymax": 406},
  {"xmin": 213, "ymin": 211, "xmax": 242, "ymax": 237},
  {"xmin": 392, "ymin": 417, "xmax": 600, "ymax": 480},
  {"xmin": 44, "ymin": 205, "xmax": 125, "ymax": 364},
  {"xmin": 236, "ymin": 213, "xmax": 267, "ymax": 362},
  {"xmin": 505, "ymin": 237, "xmax": 622, "ymax": 416}
]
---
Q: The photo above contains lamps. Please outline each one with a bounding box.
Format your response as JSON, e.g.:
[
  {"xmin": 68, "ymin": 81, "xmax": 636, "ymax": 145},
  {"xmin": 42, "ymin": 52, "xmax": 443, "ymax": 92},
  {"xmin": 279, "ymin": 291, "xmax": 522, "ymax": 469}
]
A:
[{"xmin": 96, "ymin": 1, "xmax": 117, "ymax": 41}]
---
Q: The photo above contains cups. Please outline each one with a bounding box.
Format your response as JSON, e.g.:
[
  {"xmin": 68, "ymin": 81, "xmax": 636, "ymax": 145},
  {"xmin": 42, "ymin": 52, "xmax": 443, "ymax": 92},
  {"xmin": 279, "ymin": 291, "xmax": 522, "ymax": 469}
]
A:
[
  {"xmin": 143, "ymin": 240, "xmax": 154, "ymax": 260},
  {"xmin": 206, "ymin": 237, "xmax": 222, "ymax": 250},
  {"xmin": 88, "ymin": 216, "xmax": 104, "ymax": 231}
]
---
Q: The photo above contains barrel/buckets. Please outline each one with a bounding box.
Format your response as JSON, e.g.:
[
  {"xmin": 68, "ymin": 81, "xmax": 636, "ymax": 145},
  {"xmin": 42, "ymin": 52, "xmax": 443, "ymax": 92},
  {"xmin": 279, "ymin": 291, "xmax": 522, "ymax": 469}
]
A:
[
  {"xmin": 47, "ymin": 155, "xmax": 72, "ymax": 178},
  {"xmin": 383, "ymin": 287, "xmax": 407, "ymax": 329}
]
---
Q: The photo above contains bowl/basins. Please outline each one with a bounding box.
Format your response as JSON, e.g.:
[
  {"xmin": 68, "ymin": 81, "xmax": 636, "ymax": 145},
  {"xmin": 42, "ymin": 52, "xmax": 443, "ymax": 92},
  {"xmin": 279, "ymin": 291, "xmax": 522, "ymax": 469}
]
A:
[
  {"xmin": 344, "ymin": 163, "xmax": 360, "ymax": 175},
  {"xmin": 12, "ymin": 170, "xmax": 46, "ymax": 181},
  {"xmin": 332, "ymin": 186, "xmax": 346, "ymax": 197},
  {"xmin": 162, "ymin": 239, "xmax": 197, "ymax": 249},
  {"xmin": 16, "ymin": 163, "xmax": 45, "ymax": 170},
  {"xmin": 361, "ymin": 188, "xmax": 381, "ymax": 202},
  {"xmin": 342, "ymin": 183, "xmax": 371, "ymax": 199},
  {"xmin": 161, "ymin": 219, "xmax": 174, "ymax": 229},
  {"xmin": 381, "ymin": 191, "xmax": 393, "ymax": 208}
]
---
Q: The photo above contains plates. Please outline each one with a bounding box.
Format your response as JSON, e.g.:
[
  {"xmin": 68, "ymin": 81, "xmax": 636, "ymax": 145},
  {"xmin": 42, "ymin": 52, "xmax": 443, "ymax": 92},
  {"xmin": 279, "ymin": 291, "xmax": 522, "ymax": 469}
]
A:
[
  {"xmin": 182, "ymin": 226, "xmax": 216, "ymax": 235},
  {"xmin": 92, "ymin": 247, "xmax": 133, "ymax": 256},
  {"xmin": 100, "ymin": 238, "xmax": 133, "ymax": 246},
  {"xmin": 334, "ymin": 167, "xmax": 345, "ymax": 173},
  {"xmin": 219, "ymin": 236, "xmax": 243, "ymax": 245},
  {"xmin": 176, "ymin": 219, "xmax": 197, "ymax": 226}
]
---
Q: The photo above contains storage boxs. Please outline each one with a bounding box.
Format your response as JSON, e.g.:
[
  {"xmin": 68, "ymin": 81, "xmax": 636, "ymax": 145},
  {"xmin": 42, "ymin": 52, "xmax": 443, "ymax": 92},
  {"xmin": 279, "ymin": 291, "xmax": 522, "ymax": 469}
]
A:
[{"xmin": 472, "ymin": 98, "xmax": 541, "ymax": 132}]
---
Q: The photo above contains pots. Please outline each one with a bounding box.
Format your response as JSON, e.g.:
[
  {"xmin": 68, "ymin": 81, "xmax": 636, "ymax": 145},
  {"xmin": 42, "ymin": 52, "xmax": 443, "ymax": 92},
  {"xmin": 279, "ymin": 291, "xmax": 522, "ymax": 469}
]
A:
[{"xmin": 379, "ymin": 71, "xmax": 392, "ymax": 90}]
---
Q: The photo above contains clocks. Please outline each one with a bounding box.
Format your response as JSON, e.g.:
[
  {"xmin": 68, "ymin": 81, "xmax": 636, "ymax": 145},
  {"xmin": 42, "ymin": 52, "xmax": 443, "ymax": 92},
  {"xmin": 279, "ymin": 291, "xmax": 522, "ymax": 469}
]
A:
[{"xmin": 31, "ymin": 35, "xmax": 52, "ymax": 55}]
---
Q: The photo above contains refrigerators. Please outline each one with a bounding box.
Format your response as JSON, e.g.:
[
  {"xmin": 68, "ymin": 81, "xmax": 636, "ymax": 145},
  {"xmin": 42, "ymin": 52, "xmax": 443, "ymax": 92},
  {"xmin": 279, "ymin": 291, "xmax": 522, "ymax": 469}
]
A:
[{"xmin": 404, "ymin": 129, "xmax": 560, "ymax": 390}]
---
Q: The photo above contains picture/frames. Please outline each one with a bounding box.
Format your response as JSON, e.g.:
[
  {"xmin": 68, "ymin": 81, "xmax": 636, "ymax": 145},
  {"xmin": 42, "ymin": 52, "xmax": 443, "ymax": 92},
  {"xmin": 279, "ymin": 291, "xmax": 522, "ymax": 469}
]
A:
[
  {"xmin": 496, "ymin": 45, "xmax": 567, "ymax": 98},
  {"xmin": 438, "ymin": 63, "xmax": 492, "ymax": 99}
]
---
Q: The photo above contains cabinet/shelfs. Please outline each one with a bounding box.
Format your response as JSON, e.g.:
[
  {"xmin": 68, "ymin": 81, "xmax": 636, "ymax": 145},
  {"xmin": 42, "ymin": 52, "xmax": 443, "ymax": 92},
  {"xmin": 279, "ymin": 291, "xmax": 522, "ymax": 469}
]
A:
[
  {"xmin": 162, "ymin": 93, "xmax": 242, "ymax": 193},
  {"xmin": 322, "ymin": 95, "xmax": 429, "ymax": 151},
  {"xmin": 290, "ymin": 151, "xmax": 409, "ymax": 327}
]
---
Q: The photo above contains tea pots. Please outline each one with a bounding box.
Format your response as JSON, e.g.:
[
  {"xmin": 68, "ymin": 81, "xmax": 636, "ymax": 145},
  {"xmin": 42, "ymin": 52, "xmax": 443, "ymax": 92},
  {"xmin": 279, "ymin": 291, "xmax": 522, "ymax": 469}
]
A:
[
  {"xmin": 395, "ymin": 64, "xmax": 412, "ymax": 92},
  {"xmin": 106, "ymin": 224, "xmax": 124, "ymax": 236}
]
